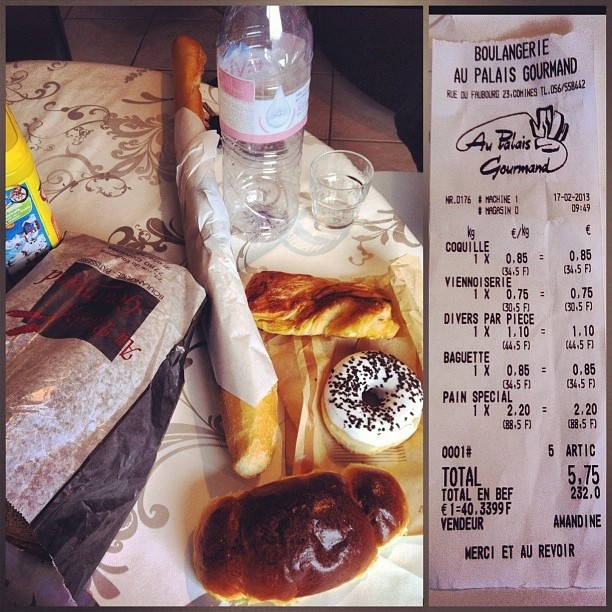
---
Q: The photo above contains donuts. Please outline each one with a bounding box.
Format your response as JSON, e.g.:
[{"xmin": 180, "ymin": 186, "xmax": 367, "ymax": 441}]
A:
[{"xmin": 319, "ymin": 348, "xmax": 423, "ymax": 457}]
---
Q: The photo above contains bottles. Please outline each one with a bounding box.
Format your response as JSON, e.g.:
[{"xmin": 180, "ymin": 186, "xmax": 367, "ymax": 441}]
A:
[{"xmin": 215, "ymin": 1, "xmax": 312, "ymax": 241}]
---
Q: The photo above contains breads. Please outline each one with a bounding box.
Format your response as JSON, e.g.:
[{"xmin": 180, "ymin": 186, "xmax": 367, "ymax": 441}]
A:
[
  {"xmin": 171, "ymin": 33, "xmax": 279, "ymax": 479},
  {"xmin": 191, "ymin": 462, "xmax": 411, "ymax": 606},
  {"xmin": 245, "ymin": 270, "xmax": 400, "ymax": 342}
]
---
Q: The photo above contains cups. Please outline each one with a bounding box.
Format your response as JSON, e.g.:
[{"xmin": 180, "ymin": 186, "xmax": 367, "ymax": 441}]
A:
[{"xmin": 310, "ymin": 151, "xmax": 373, "ymax": 227}]
